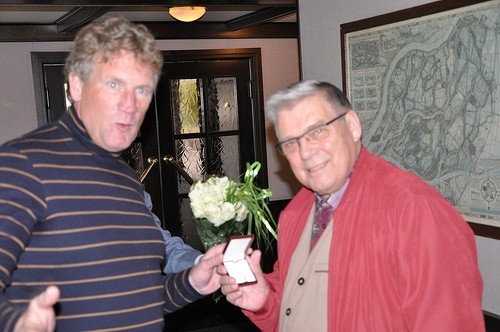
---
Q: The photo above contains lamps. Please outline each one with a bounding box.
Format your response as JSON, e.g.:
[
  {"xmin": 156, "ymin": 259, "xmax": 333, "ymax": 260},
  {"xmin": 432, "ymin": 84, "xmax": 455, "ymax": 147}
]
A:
[{"xmin": 168, "ymin": 5, "xmax": 206, "ymax": 22}]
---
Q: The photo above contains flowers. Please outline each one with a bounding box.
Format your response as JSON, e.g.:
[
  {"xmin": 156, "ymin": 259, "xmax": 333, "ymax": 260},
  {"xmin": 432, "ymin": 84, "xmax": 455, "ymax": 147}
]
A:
[{"xmin": 187, "ymin": 160, "xmax": 281, "ymax": 254}]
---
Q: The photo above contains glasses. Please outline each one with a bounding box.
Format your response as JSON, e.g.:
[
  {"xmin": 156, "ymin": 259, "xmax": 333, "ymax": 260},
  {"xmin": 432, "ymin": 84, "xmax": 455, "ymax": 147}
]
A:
[{"xmin": 275, "ymin": 112, "xmax": 347, "ymax": 156}]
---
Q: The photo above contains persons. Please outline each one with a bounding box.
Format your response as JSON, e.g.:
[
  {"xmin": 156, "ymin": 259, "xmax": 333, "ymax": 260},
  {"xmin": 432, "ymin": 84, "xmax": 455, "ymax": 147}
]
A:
[
  {"xmin": 0, "ymin": 13, "xmax": 252, "ymax": 332},
  {"xmin": 144, "ymin": 192, "xmax": 227, "ymax": 275},
  {"xmin": 216, "ymin": 80, "xmax": 486, "ymax": 332}
]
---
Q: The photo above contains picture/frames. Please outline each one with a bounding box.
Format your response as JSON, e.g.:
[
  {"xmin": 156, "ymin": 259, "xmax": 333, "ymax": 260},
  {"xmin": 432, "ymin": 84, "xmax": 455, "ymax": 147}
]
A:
[{"xmin": 339, "ymin": 0, "xmax": 500, "ymax": 242}]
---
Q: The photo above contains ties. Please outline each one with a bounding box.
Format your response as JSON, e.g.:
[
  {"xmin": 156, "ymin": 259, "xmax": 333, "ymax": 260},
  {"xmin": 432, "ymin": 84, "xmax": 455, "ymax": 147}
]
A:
[{"xmin": 309, "ymin": 204, "xmax": 331, "ymax": 252}]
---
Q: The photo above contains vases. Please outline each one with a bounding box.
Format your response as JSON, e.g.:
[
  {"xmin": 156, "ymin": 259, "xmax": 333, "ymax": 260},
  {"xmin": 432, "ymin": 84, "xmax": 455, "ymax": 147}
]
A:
[{"xmin": 190, "ymin": 215, "xmax": 250, "ymax": 255}]
